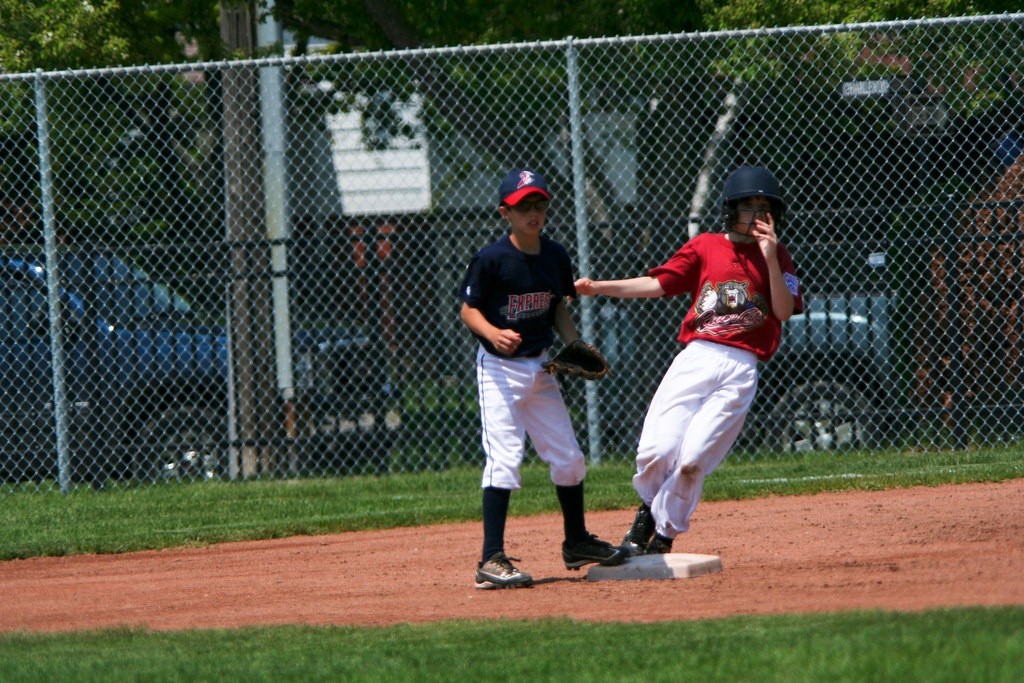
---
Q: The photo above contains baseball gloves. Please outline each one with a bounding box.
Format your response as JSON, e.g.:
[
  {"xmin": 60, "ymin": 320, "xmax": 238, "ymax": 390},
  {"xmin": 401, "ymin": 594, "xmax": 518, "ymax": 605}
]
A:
[{"xmin": 537, "ymin": 338, "xmax": 616, "ymax": 401}]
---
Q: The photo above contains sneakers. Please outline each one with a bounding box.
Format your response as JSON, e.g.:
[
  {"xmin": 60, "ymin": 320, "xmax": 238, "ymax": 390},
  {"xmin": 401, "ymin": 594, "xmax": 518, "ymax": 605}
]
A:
[
  {"xmin": 561, "ymin": 530, "xmax": 628, "ymax": 571},
  {"xmin": 622, "ymin": 507, "xmax": 655, "ymax": 552},
  {"xmin": 644, "ymin": 534, "xmax": 672, "ymax": 555},
  {"xmin": 475, "ymin": 550, "xmax": 533, "ymax": 590}
]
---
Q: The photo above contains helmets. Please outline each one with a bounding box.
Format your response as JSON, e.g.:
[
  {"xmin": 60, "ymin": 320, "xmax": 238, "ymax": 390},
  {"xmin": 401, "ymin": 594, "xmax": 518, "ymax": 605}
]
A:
[{"xmin": 719, "ymin": 165, "xmax": 785, "ymax": 225}]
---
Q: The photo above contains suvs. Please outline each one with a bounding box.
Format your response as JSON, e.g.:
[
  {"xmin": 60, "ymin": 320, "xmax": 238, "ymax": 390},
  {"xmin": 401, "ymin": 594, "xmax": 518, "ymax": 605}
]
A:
[{"xmin": 0, "ymin": 254, "xmax": 404, "ymax": 484}]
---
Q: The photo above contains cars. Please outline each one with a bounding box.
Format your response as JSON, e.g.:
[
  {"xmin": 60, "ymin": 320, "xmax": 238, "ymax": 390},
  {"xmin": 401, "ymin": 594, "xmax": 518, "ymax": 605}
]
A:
[{"xmin": 595, "ymin": 293, "xmax": 892, "ymax": 455}]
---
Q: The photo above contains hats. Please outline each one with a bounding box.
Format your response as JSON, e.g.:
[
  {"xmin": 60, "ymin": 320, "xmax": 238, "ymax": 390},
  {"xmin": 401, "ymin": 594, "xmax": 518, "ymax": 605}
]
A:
[{"xmin": 498, "ymin": 167, "xmax": 551, "ymax": 206}]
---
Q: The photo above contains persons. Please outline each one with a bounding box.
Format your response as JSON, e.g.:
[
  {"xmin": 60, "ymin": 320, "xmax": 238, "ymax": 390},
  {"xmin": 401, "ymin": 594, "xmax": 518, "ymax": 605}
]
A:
[
  {"xmin": 573, "ymin": 167, "xmax": 805, "ymax": 555},
  {"xmin": 461, "ymin": 168, "xmax": 630, "ymax": 588}
]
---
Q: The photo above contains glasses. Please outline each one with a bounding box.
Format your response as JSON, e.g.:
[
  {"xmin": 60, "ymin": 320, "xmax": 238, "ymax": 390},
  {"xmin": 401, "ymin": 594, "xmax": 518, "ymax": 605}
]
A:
[{"xmin": 512, "ymin": 198, "xmax": 549, "ymax": 212}]
